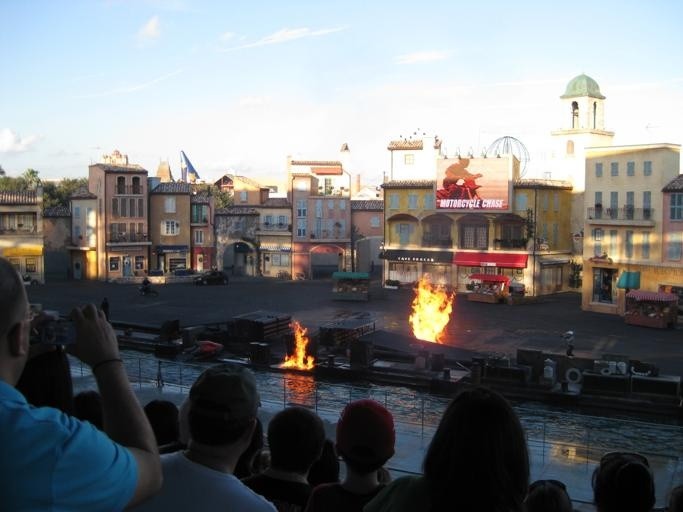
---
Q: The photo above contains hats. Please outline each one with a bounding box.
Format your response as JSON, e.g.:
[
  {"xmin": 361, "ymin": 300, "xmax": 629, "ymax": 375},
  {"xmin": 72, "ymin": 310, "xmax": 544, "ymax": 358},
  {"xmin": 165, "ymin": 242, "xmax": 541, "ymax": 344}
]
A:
[
  {"xmin": 190, "ymin": 364, "xmax": 262, "ymax": 417},
  {"xmin": 267, "ymin": 407, "xmax": 326, "ymax": 454},
  {"xmin": 335, "ymin": 400, "xmax": 396, "ymax": 461},
  {"xmin": 591, "ymin": 451, "xmax": 655, "ymax": 494}
]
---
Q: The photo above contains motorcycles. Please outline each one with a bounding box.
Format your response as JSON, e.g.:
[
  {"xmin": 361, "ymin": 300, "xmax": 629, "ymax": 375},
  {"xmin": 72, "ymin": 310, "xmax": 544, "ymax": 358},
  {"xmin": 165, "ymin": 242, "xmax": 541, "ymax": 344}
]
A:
[{"xmin": 136, "ymin": 284, "xmax": 158, "ymax": 297}]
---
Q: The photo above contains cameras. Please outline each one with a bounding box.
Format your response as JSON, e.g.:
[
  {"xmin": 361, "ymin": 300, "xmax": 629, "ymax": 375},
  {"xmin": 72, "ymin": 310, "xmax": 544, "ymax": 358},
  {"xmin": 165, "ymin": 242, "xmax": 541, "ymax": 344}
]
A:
[{"xmin": 41, "ymin": 320, "xmax": 78, "ymax": 352}]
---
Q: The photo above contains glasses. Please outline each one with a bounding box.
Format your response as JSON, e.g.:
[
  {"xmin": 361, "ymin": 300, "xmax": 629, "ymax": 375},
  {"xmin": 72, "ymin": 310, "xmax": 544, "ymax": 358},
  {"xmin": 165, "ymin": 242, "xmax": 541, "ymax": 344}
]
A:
[{"xmin": 526, "ymin": 478, "xmax": 573, "ymax": 504}]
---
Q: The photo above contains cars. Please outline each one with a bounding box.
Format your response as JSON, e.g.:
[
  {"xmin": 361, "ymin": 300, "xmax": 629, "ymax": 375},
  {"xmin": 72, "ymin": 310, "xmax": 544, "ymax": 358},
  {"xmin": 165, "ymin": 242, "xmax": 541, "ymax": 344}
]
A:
[{"xmin": 191, "ymin": 270, "xmax": 228, "ymax": 285}]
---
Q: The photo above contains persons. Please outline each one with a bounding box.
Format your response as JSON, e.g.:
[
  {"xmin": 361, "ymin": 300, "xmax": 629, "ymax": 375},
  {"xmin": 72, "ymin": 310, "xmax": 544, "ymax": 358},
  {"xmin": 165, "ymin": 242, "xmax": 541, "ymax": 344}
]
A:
[
  {"xmin": 0, "ymin": 260, "xmax": 163, "ymax": 512},
  {"xmin": 442, "ymin": 158, "xmax": 483, "ymax": 199},
  {"xmin": 143, "ymin": 364, "xmax": 683, "ymax": 512}
]
